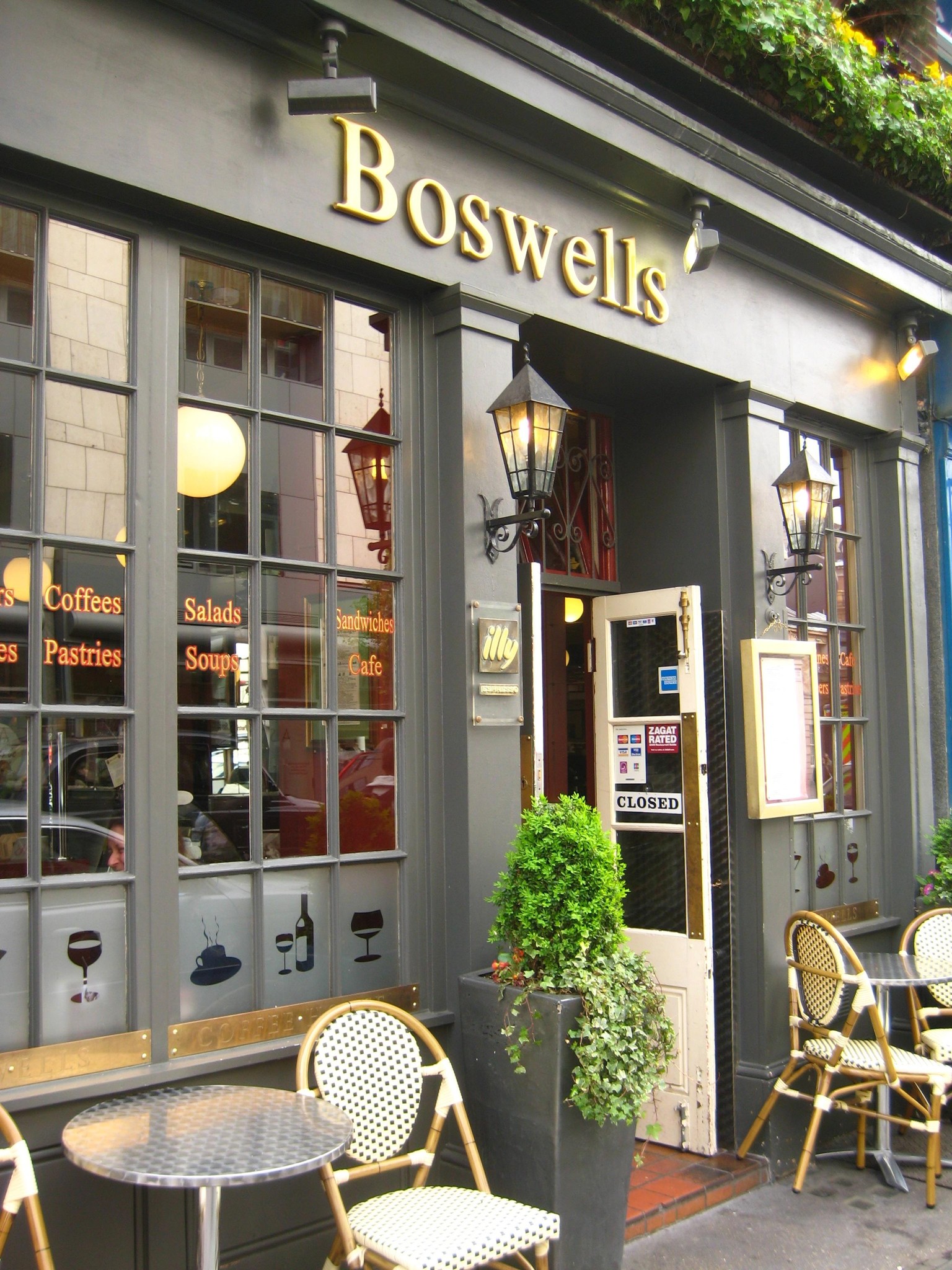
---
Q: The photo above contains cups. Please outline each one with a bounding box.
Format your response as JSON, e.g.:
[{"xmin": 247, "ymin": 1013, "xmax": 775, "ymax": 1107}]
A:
[{"xmin": 184, "ymin": 839, "xmax": 202, "ymax": 860}]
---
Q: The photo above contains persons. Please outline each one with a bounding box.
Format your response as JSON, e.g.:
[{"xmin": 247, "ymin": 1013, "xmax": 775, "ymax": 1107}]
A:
[
  {"xmin": 103, "ymin": 816, "xmax": 187, "ymax": 873},
  {"xmin": 72, "ymin": 761, "xmax": 93, "ymax": 787},
  {"xmin": 190, "ymin": 810, "xmax": 231, "ymax": 853}
]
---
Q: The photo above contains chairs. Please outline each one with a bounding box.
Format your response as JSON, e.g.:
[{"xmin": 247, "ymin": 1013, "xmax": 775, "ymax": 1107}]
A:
[
  {"xmin": 896, "ymin": 906, "xmax": 952, "ymax": 1135},
  {"xmin": 291, "ymin": 998, "xmax": 564, "ymax": 1270},
  {"xmin": 0, "ymin": 1104, "xmax": 55, "ymax": 1270},
  {"xmin": 736, "ymin": 910, "xmax": 952, "ymax": 1210}
]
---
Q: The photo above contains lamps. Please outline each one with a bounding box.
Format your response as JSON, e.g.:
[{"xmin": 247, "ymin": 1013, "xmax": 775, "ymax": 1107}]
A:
[
  {"xmin": 284, "ymin": 19, "xmax": 379, "ymax": 116},
  {"xmin": 890, "ymin": 312, "xmax": 940, "ymax": 383},
  {"xmin": 341, "ymin": 385, "xmax": 391, "ymax": 564},
  {"xmin": 477, "ymin": 340, "xmax": 575, "ymax": 563},
  {"xmin": 680, "ymin": 194, "xmax": 721, "ymax": 276},
  {"xmin": 175, "ymin": 280, "xmax": 246, "ymax": 500},
  {"xmin": 761, "ymin": 428, "xmax": 840, "ymax": 603},
  {"xmin": 114, "ymin": 523, "xmax": 128, "ymax": 568},
  {"xmin": 3, "ymin": 543, "xmax": 52, "ymax": 603},
  {"xmin": 564, "ymin": 596, "xmax": 584, "ymax": 623}
]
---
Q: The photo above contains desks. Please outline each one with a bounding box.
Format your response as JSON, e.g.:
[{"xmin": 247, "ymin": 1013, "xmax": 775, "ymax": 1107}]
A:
[
  {"xmin": 60, "ymin": 1085, "xmax": 356, "ymax": 1270},
  {"xmin": 817, "ymin": 951, "xmax": 952, "ymax": 1194}
]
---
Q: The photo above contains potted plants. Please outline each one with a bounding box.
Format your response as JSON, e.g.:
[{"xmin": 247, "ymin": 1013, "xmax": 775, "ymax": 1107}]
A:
[{"xmin": 454, "ymin": 788, "xmax": 679, "ymax": 1270}]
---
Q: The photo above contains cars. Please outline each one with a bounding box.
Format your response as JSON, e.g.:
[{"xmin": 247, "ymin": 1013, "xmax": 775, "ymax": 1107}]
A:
[
  {"xmin": 0, "ymin": 799, "xmax": 202, "ymax": 879},
  {"xmin": 231, "ymin": 733, "xmax": 394, "ymax": 808},
  {"xmin": 0, "ymin": 731, "xmax": 324, "ymax": 861}
]
